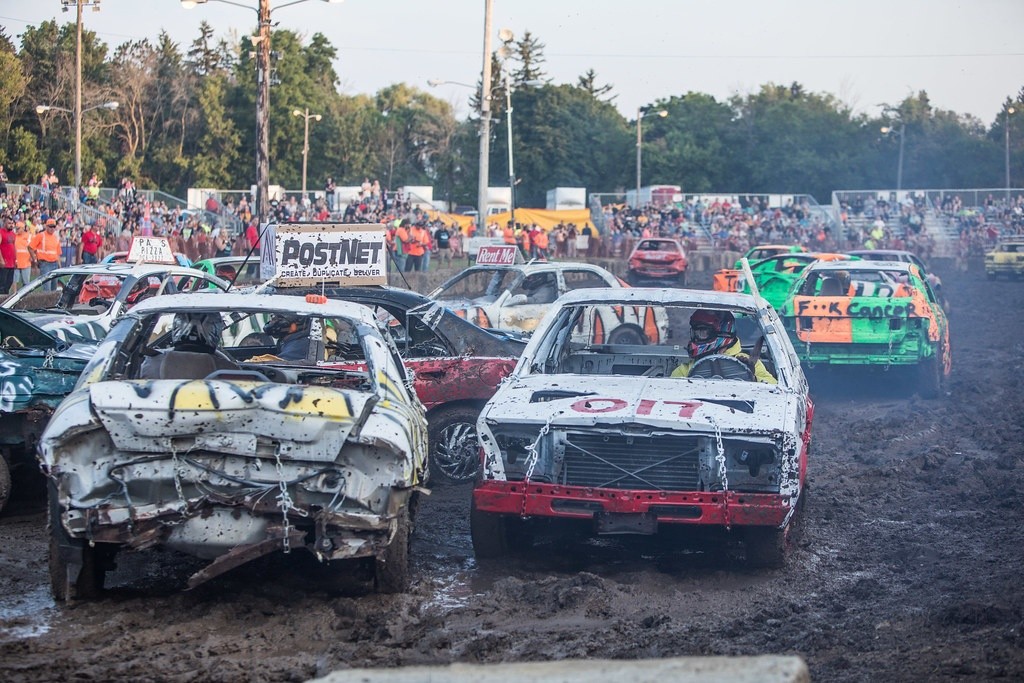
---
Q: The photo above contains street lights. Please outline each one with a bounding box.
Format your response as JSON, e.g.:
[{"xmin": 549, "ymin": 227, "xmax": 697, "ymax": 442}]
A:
[
  {"xmin": 1001, "ymin": 106, "xmax": 1016, "ymax": 205},
  {"xmin": 35, "ymin": 0, "xmax": 120, "ymax": 187},
  {"xmin": 177, "ymin": 0, "xmax": 344, "ymax": 240},
  {"xmin": 293, "ymin": 108, "xmax": 323, "ymax": 199},
  {"xmin": 496, "ymin": 27, "xmax": 515, "ymax": 212},
  {"xmin": 427, "ymin": 77, "xmax": 549, "ymax": 237},
  {"xmin": 880, "ymin": 120, "xmax": 906, "ymax": 188},
  {"xmin": 635, "ymin": 107, "xmax": 669, "ymax": 209}
]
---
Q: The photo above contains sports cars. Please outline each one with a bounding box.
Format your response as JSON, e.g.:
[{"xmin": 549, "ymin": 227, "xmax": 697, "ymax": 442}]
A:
[
  {"xmin": 627, "ymin": 237, "xmax": 689, "ymax": 287},
  {"xmin": 983, "ymin": 240, "xmax": 1024, "ymax": 279},
  {"xmin": 1, "ymin": 219, "xmax": 587, "ymax": 515},
  {"xmin": 712, "ymin": 243, "xmax": 952, "ymax": 400},
  {"xmin": 35, "ymin": 291, "xmax": 431, "ymax": 601},
  {"xmin": 468, "ymin": 286, "xmax": 814, "ymax": 570},
  {"xmin": 382, "ymin": 244, "xmax": 674, "ymax": 347}
]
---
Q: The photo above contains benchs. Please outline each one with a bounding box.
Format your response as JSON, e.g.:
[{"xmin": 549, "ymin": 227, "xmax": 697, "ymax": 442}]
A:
[
  {"xmin": 841, "ymin": 206, "xmax": 1024, "ymax": 258},
  {"xmin": 593, "ymin": 210, "xmax": 824, "ymax": 259}
]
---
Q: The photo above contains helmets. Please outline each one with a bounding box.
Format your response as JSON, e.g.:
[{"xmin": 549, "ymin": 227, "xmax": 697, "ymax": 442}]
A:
[
  {"xmin": 523, "ymin": 271, "xmax": 548, "ymax": 291},
  {"xmin": 649, "ymin": 241, "xmax": 659, "ymax": 250},
  {"xmin": 171, "ymin": 312, "xmax": 223, "ymax": 354},
  {"xmin": 264, "ymin": 312, "xmax": 311, "ymax": 339},
  {"xmin": 215, "ymin": 265, "xmax": 236, "ymax": 286},
  {"xmin": 115, "ymin": 274, "xmax": 150, "ymax": 304},
  {"xmin": 686, "ymin": 309, "xmax": 736, "ymax": 359},
  {"xmin": 822, "ymin": 270, "xmax": 851, "ymax": 290}
]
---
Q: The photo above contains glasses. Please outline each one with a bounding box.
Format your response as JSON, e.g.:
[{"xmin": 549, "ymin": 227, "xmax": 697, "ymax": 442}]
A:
[
  {"xmin": 690, "ymin": 326, "xmax": 714, "ymax": 341},
  {"xmin": 47, "ymin": 224, "xmax": 56, "ymax": 228},
  {"xmin": 15, "ymin": 226, "xmax": 20, "ymax": 230}
]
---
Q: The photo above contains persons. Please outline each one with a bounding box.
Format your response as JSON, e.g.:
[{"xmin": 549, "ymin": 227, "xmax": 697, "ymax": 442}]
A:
[
  {"xmin": 0, "ymin": 166, "xmax": 592, "ymax": 295},
  {"xmin": 834, "ymin": 271, "xmax": 850, "ymax": 293},
  {"xmin": 264, "ymin": 311, "xmax": 326, "ymax": 361},
  {"xmin": 516, "ymin": 273, "xmax": 555, "ymax": 303},
  {"xmin": 669, "ymin": 308, "xmax": 776, "ymax": 384},
  {"xmin": 215, "ymin": 265, "xmax": 236, "ymax": 282},
  {"xmin": 141, "ymin": 310, "xmax": 230, "ymax": 360},
  {"xmin": 605, "ymin": 192, "xmax": 1024, "ymax": 273}
]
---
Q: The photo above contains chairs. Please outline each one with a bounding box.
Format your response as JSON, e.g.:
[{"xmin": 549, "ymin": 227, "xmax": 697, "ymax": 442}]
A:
[
  {"xmin": 149, "ymin": 288, "xmax": 166, "ymax": 295},
  {"xmin": 161, "ymin": 350, "xmax": 219, "ymax": 381},
  {"xmin": 821, "ymin": 278, "xmax": 843, "ymax": 295}
]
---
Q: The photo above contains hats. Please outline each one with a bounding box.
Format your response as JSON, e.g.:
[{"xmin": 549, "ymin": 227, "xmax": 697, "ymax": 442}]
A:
[
  {"xmin": 46, "ymin": 219, "xmax": 57, "ymax": 225},
  {"xmin": 92, "ymin": 219, "xmax": 102, "ymax": 226},
  {"xmin": 15, "ymin": 222, "xmax": 24, "ymax": 228}
]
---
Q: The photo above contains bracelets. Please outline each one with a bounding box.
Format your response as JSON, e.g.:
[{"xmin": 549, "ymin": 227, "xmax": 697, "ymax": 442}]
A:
[{"xmin": 15, "ymin": 260, "xmax": 17, "ymax": 262}]
